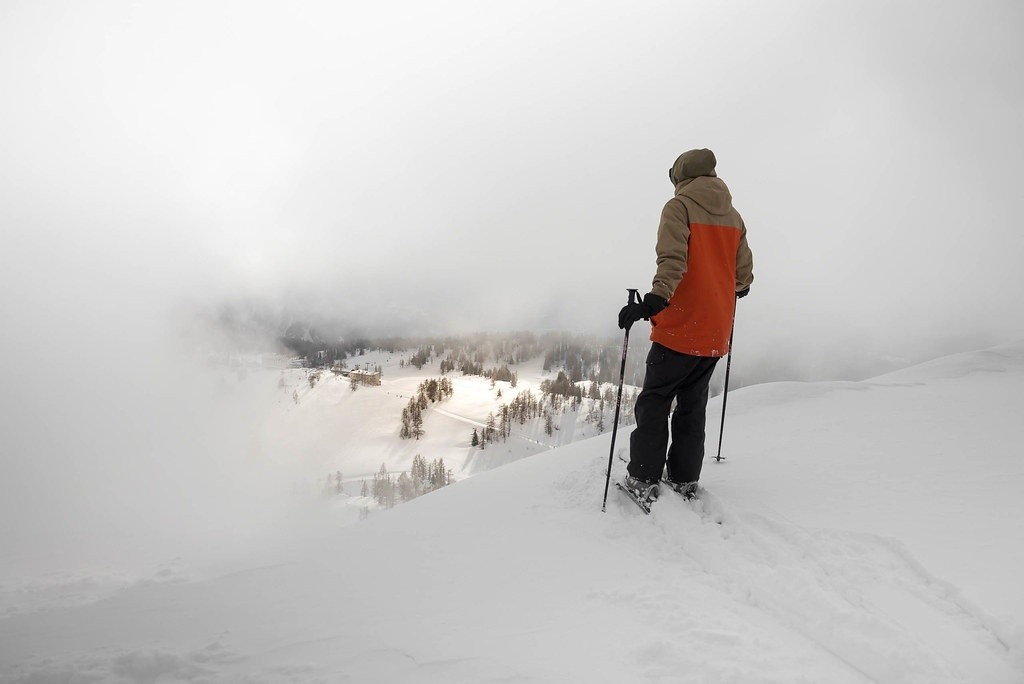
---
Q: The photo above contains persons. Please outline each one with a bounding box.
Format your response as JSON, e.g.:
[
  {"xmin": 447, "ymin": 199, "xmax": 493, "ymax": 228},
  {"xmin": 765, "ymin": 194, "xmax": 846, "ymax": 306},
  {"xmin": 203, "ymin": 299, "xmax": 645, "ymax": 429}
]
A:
[{"xmin": 618, "ymin": 148, "xmax": 754, "ymax": 501}]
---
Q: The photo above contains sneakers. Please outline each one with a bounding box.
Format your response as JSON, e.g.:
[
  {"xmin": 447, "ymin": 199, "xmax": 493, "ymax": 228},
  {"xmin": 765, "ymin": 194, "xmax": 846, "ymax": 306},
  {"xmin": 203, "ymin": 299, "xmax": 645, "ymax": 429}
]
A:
[
  {"xmin": 662, "ymin": 468, "xmax": 697, "ymax": 494},
  {"xmin": 625, "ymin": 473, "xmax": 657, "ymax": 502}
]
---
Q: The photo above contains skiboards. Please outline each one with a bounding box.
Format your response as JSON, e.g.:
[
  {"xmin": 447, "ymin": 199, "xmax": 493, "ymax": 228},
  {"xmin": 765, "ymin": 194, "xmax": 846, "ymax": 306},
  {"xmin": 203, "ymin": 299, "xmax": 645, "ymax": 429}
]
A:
[{"xmin": 604, "ymin": 444, "xmax": 724, "ymax": 526}]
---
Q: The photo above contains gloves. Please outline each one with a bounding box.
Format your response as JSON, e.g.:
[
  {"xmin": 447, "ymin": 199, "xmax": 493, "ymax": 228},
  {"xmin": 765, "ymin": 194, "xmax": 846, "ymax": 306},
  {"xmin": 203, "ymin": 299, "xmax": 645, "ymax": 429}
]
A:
[{"xmin": 618, "ymin": 302, "xmax": 655, "ymax": 329}]
